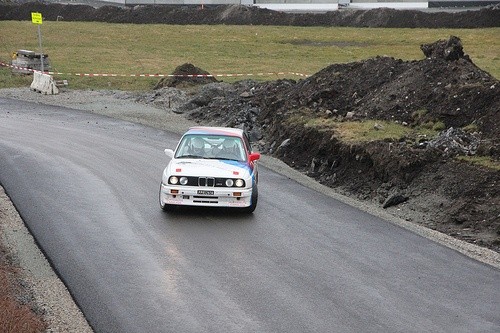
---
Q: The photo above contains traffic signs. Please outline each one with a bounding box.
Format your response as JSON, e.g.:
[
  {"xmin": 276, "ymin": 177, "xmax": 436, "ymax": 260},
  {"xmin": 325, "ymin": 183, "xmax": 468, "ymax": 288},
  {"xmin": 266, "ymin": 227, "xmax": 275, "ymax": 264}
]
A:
[{"xmin": 31, "ymin": 12, "xmax": 42, "ymax": 24}]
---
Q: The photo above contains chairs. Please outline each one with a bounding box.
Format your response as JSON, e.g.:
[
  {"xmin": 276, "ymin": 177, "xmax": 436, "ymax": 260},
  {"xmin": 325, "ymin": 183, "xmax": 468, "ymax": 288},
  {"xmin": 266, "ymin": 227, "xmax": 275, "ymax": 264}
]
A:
[
  {"xmin": 191, "ymin": 138, "xmax": 205, "ymax": 156},
  {"xmin": 223, "ymin": 138, "xmax": 240, "ymax": 158}
]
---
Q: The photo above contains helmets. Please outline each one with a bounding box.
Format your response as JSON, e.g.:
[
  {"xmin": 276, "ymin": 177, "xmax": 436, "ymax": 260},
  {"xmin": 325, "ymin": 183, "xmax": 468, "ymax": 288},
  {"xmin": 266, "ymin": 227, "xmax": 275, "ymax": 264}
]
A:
[
  {"xmin": 191, "ymin": 138, "xmax": 204, "ymax": 152},
  {"xmin": 222, "ymin": 140, "xmax": 236, "ymax": 153}
]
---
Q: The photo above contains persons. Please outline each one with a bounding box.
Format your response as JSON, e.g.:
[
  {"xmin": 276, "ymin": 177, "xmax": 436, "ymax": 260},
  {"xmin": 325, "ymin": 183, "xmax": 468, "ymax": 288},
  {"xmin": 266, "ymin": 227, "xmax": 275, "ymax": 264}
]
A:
[
  {"xmin": 221, "ymin": 140, "xmax": 239, "ymax": 158},
  {"xmin": 187, "ymin": 139, "xmax": 205, "ymax": 156}
]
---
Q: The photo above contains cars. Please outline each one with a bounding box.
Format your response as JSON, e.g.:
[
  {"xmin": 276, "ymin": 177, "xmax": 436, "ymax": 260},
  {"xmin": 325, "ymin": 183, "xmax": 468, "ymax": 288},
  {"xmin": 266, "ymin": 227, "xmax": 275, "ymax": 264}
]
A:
[{"xmin": 159, "ymin": 126, "xmax": 261, "ymax": 215}]
